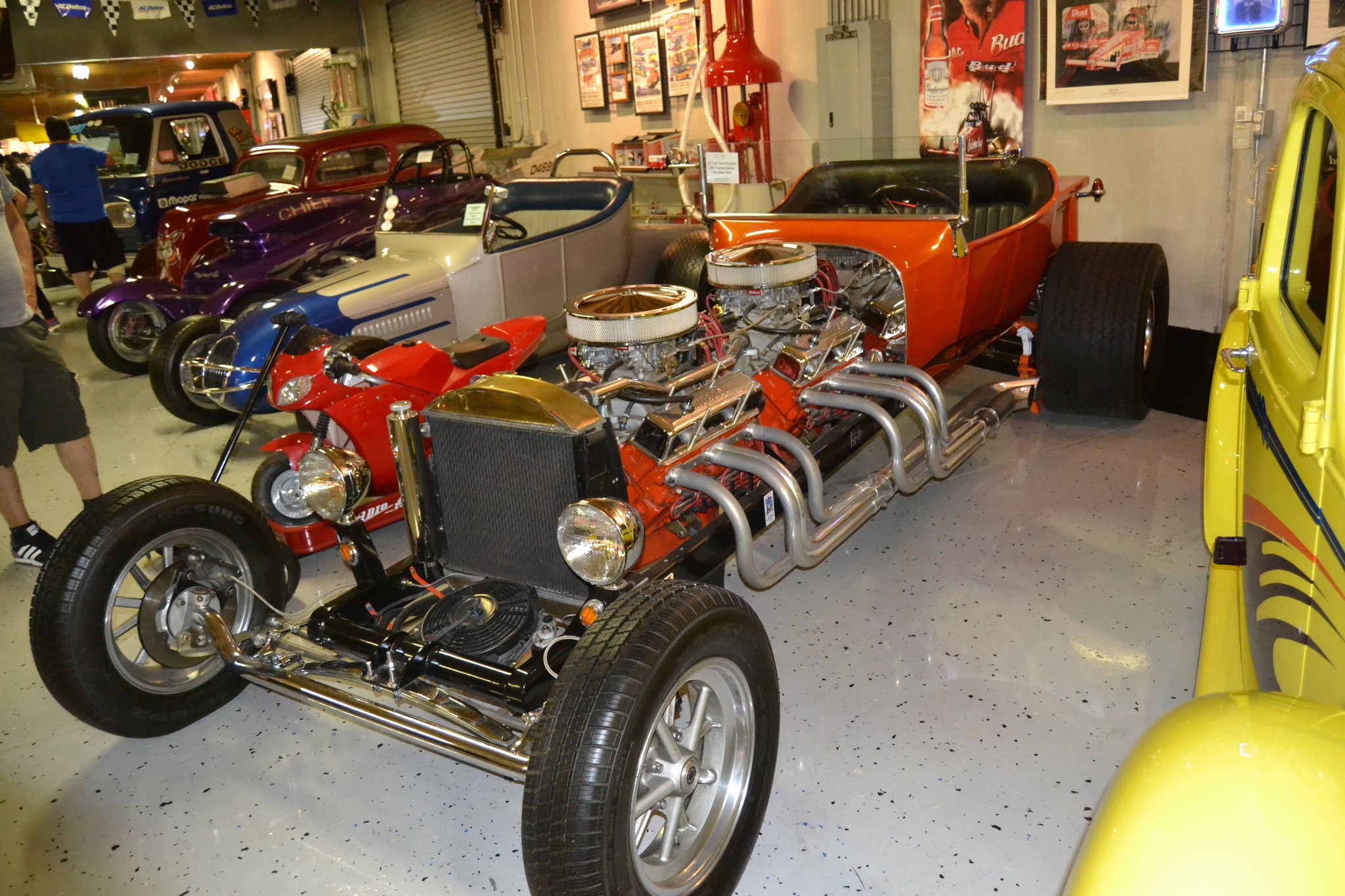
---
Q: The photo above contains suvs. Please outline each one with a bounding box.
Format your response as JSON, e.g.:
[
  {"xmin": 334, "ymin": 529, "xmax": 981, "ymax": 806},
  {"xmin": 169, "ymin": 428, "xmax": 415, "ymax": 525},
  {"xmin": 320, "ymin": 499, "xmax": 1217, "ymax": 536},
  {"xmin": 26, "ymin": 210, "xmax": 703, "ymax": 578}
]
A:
[{"xmin": 1050, "ymin": 35, "xmax": 1343, "ymax": 895}]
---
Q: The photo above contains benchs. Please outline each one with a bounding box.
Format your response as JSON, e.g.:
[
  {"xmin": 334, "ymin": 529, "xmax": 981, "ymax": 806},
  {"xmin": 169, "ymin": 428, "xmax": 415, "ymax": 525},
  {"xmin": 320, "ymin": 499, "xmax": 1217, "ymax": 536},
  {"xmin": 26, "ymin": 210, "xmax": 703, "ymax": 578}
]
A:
[{"xmin": 769, "ymin": 151, "xmax": 1053, "ymax": 244}]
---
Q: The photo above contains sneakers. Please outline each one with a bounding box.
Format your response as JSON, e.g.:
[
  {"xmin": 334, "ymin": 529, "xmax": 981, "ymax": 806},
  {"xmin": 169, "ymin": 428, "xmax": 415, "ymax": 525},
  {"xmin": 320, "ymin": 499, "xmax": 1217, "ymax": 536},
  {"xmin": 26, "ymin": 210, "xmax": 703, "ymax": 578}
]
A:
[
  {"xmin": 44, "ymin": 316, "xmax": 60, "ymax": 333},
  {"xmin": 8, "ymin": 529, "xmax": 58, "ymax": 566}
]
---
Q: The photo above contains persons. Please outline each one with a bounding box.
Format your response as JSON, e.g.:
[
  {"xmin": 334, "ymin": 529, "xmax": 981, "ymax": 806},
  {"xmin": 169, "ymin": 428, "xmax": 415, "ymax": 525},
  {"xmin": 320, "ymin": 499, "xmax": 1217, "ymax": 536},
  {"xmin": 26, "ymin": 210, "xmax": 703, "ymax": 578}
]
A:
[
  {"xmin": 948, "ymin": 0, "xmax": 1024, "ymax": 108},
  {"xmin": 0, "ymin": 152, "xmax": 33, "ymax": 201},
  {"xmin": 0, "ymin": 170, "xmax": 109, "ymax": 565},
  {"xmin": 30, "ymin": 115, "xmax": 128, "ymax": 301}
]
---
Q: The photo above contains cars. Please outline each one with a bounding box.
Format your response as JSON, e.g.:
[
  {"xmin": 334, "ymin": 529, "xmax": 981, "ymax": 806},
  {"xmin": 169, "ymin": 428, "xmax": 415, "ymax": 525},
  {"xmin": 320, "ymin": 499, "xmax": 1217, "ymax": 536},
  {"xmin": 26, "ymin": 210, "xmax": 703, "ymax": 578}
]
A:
[{"xmin": 28, "ymin": 122, "xmax": 1174, "ymax": 895}]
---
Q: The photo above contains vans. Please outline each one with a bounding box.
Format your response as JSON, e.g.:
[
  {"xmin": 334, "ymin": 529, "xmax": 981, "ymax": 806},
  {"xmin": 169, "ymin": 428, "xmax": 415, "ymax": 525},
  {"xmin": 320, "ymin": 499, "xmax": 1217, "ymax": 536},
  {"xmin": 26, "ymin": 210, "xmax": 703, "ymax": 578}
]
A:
[{"xmin": 64, "ymin": 97, "xmax": 259, "ymax": 264}]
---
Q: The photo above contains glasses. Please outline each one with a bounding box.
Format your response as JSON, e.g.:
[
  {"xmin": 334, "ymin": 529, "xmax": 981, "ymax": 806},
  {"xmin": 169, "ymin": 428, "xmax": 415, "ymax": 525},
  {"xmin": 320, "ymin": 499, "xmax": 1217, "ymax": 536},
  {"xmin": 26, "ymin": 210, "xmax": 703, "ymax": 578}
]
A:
[
  {"xmin": 1078, "ymin": 21, "xmax": 1090, "ymax": 30},
  {"xmin": 1127, "ymin": 20, "xmax": 1138, "ymax": 27}
]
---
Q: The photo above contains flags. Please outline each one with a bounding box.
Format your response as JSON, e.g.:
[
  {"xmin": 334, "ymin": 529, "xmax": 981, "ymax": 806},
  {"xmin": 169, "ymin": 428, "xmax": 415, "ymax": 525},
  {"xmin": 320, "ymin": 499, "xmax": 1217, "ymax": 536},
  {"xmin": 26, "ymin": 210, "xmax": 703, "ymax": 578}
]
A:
[
  {"xmin": 202, "ymin": 0, "xmax": 238, "ymax": 18},
  {"xmin": 53, "ymin": 0, "xmax": 93, "ymax": 19},
  {"xmin": 267, "ymin": 1, "xmax": 297, "ymax": 10},
  {"xmin": 131, "ymin": 1, "xmax": 172, "ymax": 21}
]
